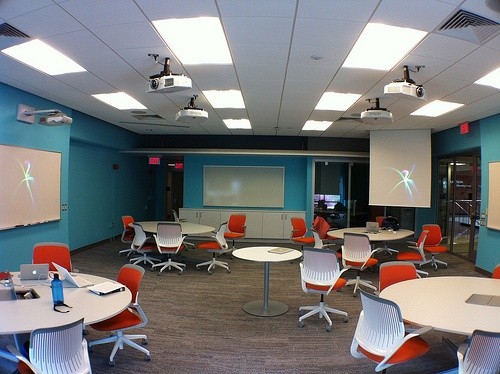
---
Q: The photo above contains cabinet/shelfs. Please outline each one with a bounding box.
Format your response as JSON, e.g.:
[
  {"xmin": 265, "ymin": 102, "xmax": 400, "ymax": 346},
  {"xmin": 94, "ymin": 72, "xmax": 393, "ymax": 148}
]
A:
[
  {"xmin": 179, "ymin": 208, "xmax": 220, "ymax": 240},
  {"xmin": 220, "ymin": 209, "xmax": 262, "ymax": 242},
  {"xmin": 262, "ymin": 210, "xmax": 306, "ymax": 243}
]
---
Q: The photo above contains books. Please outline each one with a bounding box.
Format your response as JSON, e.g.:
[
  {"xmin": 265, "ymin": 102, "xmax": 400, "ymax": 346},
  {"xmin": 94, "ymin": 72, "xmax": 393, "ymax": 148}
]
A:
[{"xmin": 89, "ymin": 287, "xmax": 126, "ymax": 296}]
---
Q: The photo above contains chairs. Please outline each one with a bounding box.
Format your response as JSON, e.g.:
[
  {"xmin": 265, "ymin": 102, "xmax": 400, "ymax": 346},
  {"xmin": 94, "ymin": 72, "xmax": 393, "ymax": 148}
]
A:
[
  {"xmin": 119, "ymin": 210, "xmax": 247, "ymax": 275},
  {"xmin": 290, "ymin": 216, "xmax": 500, "ymax": 374},
  {"xmin": 0, "ymin": 242, "xmax": 151, "ymax": 374}
]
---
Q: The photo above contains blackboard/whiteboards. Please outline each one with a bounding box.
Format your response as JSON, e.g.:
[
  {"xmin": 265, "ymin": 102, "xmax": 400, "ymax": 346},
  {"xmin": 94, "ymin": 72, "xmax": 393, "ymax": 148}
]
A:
[
  {"xmin": 202, "ymin": 164, "xmax": 285, "ymax": 208},
  {"xmin": 0, "ymin": 143, "xmax": 62, "ymax": 231}
]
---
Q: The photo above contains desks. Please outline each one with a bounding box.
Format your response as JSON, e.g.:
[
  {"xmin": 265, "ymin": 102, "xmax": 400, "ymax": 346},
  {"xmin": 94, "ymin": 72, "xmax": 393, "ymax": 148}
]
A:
[
  {"xmin": 135, "ymin": 221, "xmax": 215, "ymax": 235},
  {"xmin": 1, "ymin": 272, "xmax": 132, "ymax": 335},
  {"xmin": 232, "ymin": 246, "xmax": 303, "ymax": 316},
  {"xmin": 379, "ymin": 276, "xmax": 500, "ymax": 355},
  {"xmin": 327, "ymin": 227, "xmax": 414, "ymax": 244}
]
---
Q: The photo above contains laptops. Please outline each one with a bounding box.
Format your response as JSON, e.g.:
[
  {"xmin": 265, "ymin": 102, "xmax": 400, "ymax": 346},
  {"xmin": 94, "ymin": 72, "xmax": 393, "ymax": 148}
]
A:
[
  {"xmin": 18, "ymin": 263, "xmax": 49, "ymax": 280},
  {"xmin": 364, "ymin": 222, "xmax": 381, "ymax": 234},
  {"xmin": 52, "ymin": 262, "xmax": 94, "ymax": 288}
]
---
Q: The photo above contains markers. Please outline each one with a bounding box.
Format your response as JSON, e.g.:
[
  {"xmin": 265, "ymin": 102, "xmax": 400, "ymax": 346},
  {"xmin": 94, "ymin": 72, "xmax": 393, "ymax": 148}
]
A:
[{"xmin": 16, "ymin": 225, "xmax": 24, "ymax": 227}]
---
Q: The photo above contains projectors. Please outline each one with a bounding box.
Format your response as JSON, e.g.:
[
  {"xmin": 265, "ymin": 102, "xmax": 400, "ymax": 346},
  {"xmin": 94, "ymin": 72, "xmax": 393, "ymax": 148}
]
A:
[
  {"xmin": 383, "ymin": 81, "xmax": 425, "ymax": 100},
  {"xmin": 179, "ymin": 106, "xmax": 209, "ymax": 122},
  {"xmin": 362, "ymin": 108, "xmax": 393, "ymax": 124},
  {"xmin": 146, "ymin": 73, "xmax": 193, "ymax": 93},
  {"xmin": 39, "ymin": 114, "xmax": 73, "ymax": 125}
]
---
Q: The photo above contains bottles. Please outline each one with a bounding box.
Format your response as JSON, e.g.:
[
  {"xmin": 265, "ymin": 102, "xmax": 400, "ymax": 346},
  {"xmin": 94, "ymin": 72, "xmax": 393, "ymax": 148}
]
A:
[{"xmin": 51, "ymin": 274, "xmax": 63, "ymax": 306}]
